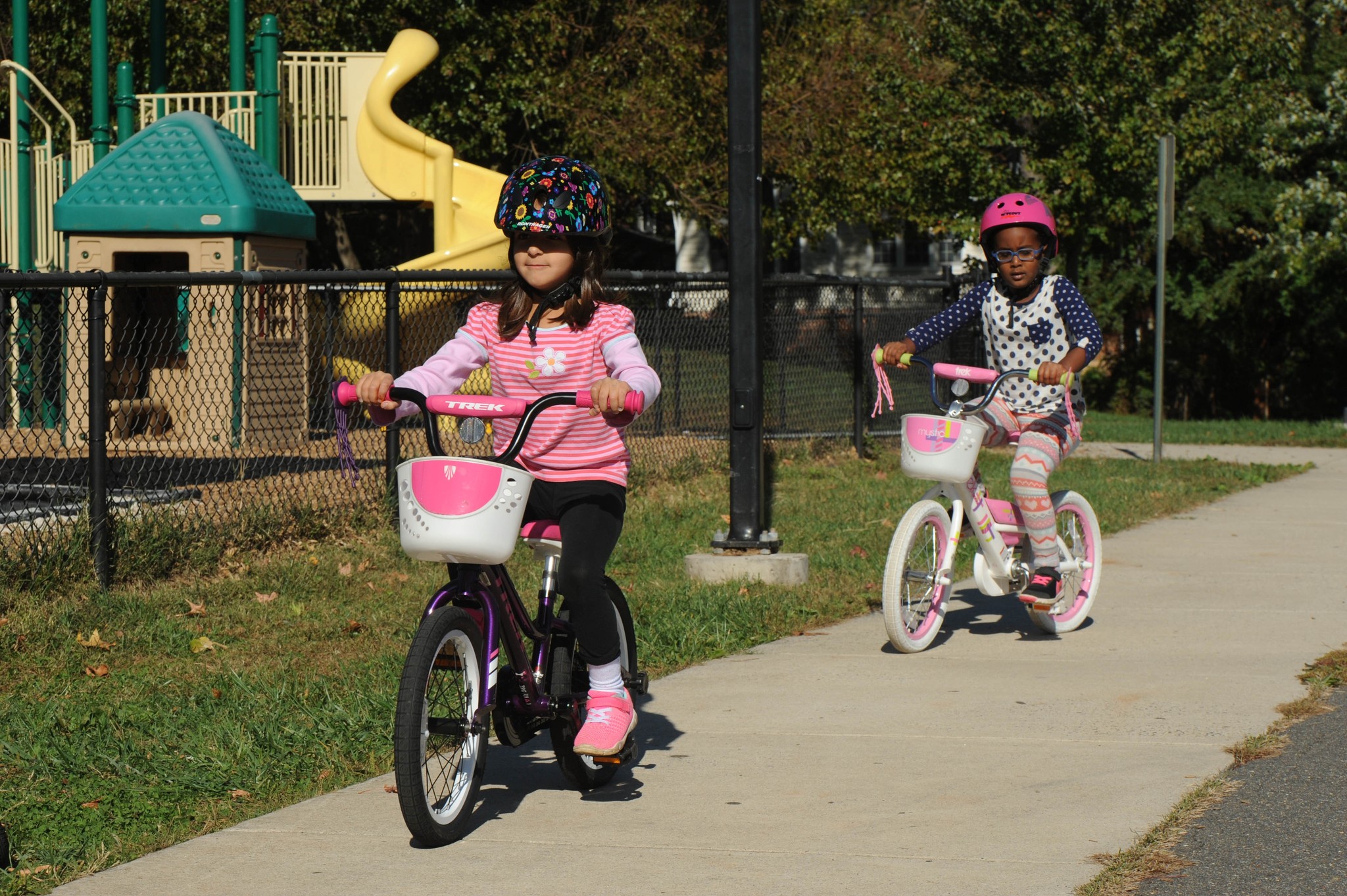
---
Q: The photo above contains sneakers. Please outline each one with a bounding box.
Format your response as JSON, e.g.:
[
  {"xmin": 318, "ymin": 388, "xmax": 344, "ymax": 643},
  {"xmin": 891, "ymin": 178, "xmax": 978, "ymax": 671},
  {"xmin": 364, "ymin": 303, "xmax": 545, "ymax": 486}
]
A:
[
  {"xmin": 573, "ymin": 690, "xmax": 638, "ymax": 756},
  {"xmin": 1019, "ymin": 567, "xmax": 1066, "ymax": 606},
  {"xmin": 947, "ymin": 489, "xmax": 989, "ymax": 532},
  {"xmin": 434, "ymin": 608, "xmax": 487, "ymax": 670}
]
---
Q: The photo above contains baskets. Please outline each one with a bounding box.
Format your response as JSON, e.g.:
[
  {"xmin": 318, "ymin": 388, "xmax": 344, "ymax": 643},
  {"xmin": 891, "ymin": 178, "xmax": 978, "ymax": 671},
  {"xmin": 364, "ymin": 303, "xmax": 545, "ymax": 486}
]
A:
[
  {"xmin": 397, "ymin": 456, "xmax": 537, "ymax": 565},
  {"xmin": 900, "ymin": 414, "xmax": 990, "ymax": 485}
]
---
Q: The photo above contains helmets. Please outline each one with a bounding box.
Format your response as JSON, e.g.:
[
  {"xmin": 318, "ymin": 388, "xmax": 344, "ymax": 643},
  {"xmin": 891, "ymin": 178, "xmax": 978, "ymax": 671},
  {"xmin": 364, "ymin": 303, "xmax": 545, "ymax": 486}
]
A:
[
  {"xmin": 979, "ymin": 192, "xmax": 1059, "ymax": 262},
  {"xmin": 494, "ymin": 157, "xmax": 612, "ymax": 242}
]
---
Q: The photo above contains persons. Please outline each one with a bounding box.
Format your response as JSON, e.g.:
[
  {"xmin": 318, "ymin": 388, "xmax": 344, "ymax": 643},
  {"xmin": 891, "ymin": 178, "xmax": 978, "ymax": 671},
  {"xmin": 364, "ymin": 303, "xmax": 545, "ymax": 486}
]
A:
[
  {"xmin": 882, "ymin": 192, "xmax": 1104, "ymax": 606},
  {"xmin": 356, "ymin": 156, "xmax": 662, "ymax": 754}
]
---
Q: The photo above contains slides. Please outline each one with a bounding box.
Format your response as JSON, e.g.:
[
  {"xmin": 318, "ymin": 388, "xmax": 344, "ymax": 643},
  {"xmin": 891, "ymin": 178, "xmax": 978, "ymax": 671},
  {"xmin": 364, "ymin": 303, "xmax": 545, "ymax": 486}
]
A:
[{"xmin": 316, "ymin": 29, "xmax": 513, "ymax": 442}]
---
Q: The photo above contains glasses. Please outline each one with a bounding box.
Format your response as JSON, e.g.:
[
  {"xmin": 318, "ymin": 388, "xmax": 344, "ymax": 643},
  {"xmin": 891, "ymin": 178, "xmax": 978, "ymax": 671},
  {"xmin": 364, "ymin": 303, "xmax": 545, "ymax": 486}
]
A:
[{"xmin": 993, "ymin": 245, "xmax": 1046, "ymax": 265}]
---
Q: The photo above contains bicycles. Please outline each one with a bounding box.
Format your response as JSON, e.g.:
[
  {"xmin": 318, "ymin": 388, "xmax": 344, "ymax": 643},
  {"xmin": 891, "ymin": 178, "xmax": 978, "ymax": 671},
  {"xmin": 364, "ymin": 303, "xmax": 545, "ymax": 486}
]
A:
[
  {"xmin": 330, "ymin": 381, "xmax": 651, "ymax": 846},
  {"xmin": 870, "ymin": 344, "xmax": 1103, "ymax": 654}
]
